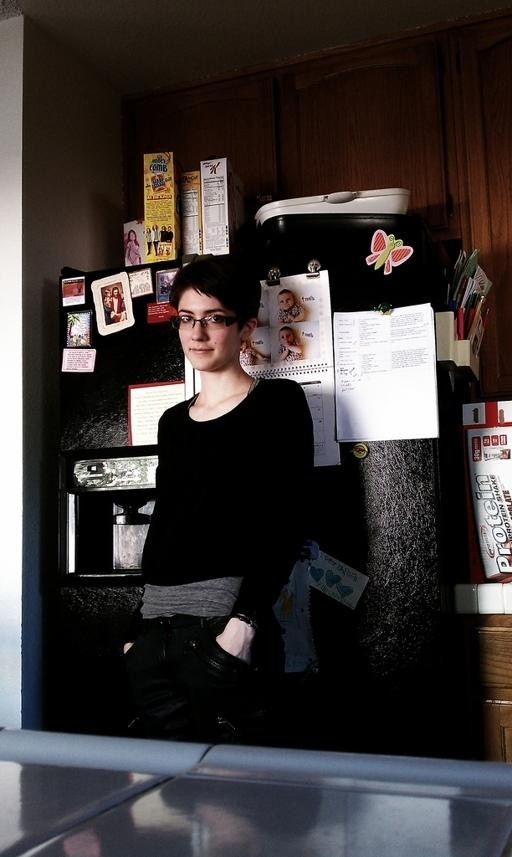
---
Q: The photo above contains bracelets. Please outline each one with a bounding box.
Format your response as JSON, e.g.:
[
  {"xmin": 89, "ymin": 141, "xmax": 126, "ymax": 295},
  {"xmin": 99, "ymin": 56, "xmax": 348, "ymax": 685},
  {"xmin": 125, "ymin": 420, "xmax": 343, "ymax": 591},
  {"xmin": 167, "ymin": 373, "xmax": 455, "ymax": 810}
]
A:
[{"xmin": 231, "ymin": 613, "xmax": 257, "ymax": 630}]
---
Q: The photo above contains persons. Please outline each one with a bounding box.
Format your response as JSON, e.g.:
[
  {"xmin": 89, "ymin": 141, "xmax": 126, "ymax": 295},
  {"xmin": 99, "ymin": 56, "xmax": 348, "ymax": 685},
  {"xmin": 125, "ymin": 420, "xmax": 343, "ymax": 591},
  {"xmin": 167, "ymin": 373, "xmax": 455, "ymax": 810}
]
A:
[
  {"xmin": 239, "ymin": 339, "xmax": 269, "ymax": 366},
  {"xmin": 104, "ymin": 288, "xmax": 112, "ymax": 317},
  {"xmin": 159, "ymin": 225, "xmax": 168, "ymax": 256},
  {"xmin": 113, "ymin": 254, "xmax": 315, "ymax": 746},
  {"xmin": 276, "ymin": 288, "xmax": 304, "ymax": 325},
  {"xmin": 145, "ymin": 228, "xmax": 152, "ymax": 255},
  {"xmin": 167, "ymin": 226, "xmax": 173, "ymax": 255},
  {"xmin": 125, "ymin": 228, "xmax": 142, "ymax": 265},
  {"xmin": 278, "ymin": 325, "xmax": 305, "ymax": 362},
  {"xmin": 151, "ymin": 224, "xmax": 161, "ymax": 256},
  {"xmin": 109, "ymin": 286, "xmax": 128, "ymax": 322}
]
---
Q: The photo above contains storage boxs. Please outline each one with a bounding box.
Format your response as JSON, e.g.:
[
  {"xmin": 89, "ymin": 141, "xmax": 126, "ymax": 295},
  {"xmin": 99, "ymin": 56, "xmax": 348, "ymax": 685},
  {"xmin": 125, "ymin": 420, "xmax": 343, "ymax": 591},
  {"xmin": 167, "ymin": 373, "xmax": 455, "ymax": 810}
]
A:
[{"xmin": 254, "ymin": 184, "xmax": 412, "ymax": 314}]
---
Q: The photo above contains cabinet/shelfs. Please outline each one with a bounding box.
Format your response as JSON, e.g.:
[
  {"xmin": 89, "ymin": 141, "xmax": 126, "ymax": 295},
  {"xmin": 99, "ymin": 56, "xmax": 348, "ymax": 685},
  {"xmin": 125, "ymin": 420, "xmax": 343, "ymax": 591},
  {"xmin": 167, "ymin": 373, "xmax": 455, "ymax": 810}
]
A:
[{"xmin": 38, "ymin": 360, "xmax": 511, "ymax": 765}]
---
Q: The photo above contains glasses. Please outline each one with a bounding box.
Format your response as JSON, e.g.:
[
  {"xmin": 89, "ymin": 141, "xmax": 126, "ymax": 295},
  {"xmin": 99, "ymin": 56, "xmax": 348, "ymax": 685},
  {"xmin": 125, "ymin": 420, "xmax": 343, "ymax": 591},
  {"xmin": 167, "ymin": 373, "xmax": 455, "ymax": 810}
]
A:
[{"xmin": 170, "ymin": 314, "xmax": 237, "ymax": 331}]
